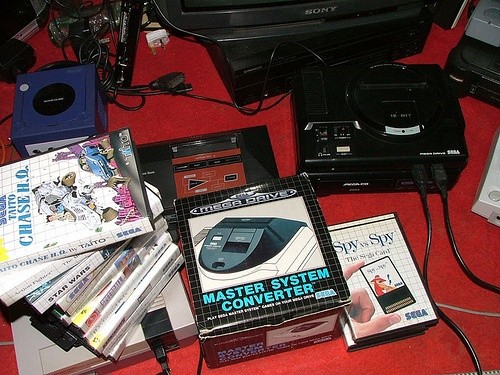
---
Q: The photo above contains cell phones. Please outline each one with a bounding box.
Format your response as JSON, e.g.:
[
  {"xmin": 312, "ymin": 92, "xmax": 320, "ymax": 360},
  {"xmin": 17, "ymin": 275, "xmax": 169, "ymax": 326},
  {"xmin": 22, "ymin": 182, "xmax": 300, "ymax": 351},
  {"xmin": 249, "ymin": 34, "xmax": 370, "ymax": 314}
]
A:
[{"xmin": 355, "ymin": 255, "xmax": 419, "ymax": 317}]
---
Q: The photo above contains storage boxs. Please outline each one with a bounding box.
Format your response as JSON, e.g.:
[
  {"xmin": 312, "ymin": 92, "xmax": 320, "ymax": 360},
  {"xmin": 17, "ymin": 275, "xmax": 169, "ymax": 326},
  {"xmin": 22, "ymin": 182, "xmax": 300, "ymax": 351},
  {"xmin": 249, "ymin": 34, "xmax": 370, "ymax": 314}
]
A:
[{"xmin": 170, "ymin": 168, "xmax": 353, "ymax": 368}]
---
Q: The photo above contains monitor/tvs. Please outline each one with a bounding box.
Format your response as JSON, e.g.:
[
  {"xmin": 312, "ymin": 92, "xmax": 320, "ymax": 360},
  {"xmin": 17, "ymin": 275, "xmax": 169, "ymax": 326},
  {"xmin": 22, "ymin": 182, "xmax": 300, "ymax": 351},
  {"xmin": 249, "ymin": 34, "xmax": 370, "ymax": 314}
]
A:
[{"xmin": 166, "ymin": 0, "xmax": 433, "ymax": 27}]
